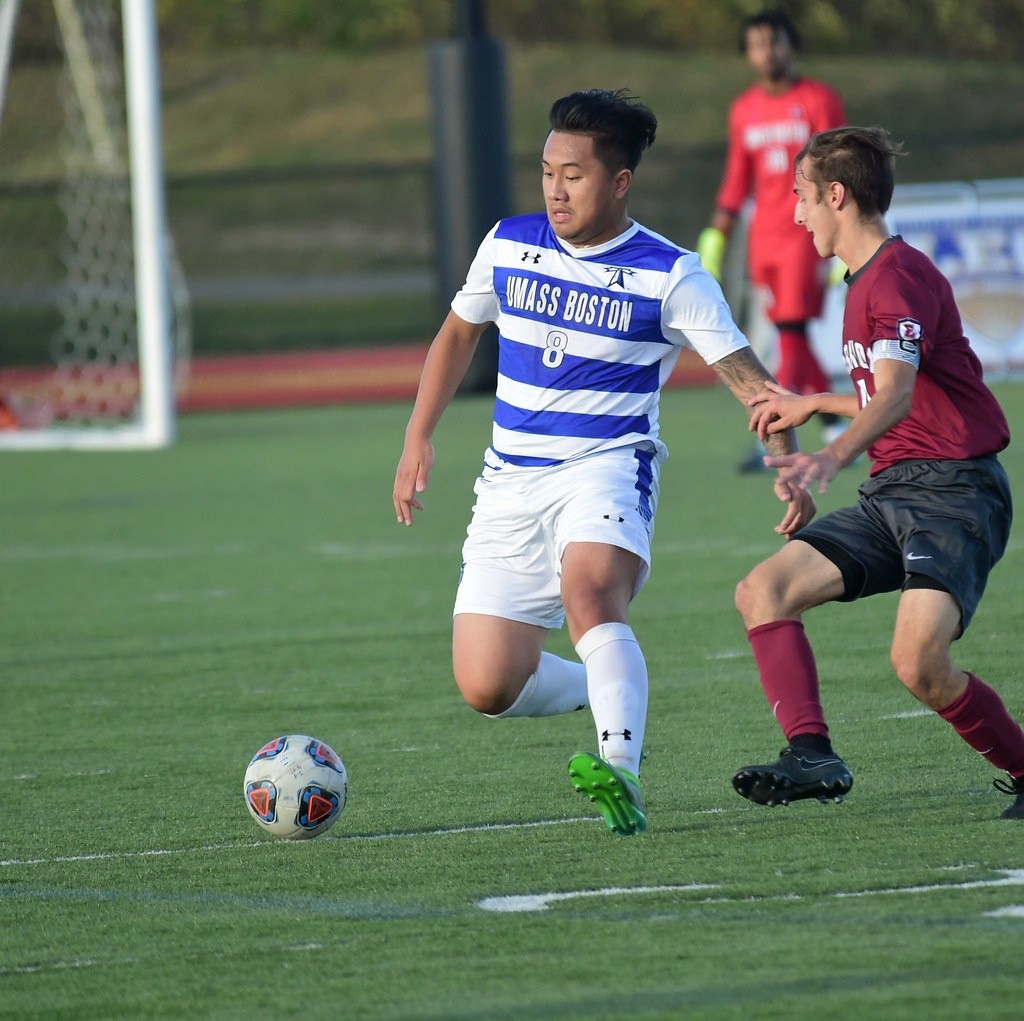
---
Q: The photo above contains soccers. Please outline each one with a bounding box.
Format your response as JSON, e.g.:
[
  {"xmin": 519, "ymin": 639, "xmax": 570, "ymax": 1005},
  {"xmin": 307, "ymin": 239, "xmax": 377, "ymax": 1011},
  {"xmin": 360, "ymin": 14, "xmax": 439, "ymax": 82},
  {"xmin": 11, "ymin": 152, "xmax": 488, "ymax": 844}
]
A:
[{"xmin": 243, "ymin": 733, "xmax": 350, "ymax": 840}]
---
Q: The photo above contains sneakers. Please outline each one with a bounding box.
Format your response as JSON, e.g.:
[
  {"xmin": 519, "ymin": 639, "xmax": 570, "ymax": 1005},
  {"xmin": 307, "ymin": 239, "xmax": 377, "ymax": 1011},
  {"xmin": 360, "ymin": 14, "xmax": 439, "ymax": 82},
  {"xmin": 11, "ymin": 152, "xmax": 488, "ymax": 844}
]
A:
[
  {"xmin": 992, "ymin": 773, "xmax": 1024, "ymax": 820},
  {"xmin": 731, "ymin": 746, "xmax": 853, "ymax": 808},
  {"xmin": 567, "ymin": 752, "xmax": 647, "ymax": 836}
]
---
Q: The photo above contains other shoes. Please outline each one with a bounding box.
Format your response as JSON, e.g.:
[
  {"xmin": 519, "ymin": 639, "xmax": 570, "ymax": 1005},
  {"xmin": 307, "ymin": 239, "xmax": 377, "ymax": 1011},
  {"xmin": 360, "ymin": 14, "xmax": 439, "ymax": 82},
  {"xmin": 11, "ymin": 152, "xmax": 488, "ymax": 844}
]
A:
[
  {"xmin": 823, "ymin": 427, "xmax": 859, "ymax": 466},
  {"xmin": 737, "ymin": 452, "xmax": 777, "ymax": 473}
]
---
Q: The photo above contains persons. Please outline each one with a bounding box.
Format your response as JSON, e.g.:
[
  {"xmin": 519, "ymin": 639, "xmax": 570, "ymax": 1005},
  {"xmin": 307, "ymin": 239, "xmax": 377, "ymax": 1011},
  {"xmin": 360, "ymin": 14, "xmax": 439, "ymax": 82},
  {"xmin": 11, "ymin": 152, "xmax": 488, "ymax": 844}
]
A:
[
  {"xmin": 731, "ymin": 128, "xmax": 1024, "ymax": 819},
  {"xmin": 393, "ymin": 89, "xmax": 817, "ymax": 835},
  {"xmin": 697, "ymin": 10, "xmax": 855, "ymax": 475}
]
